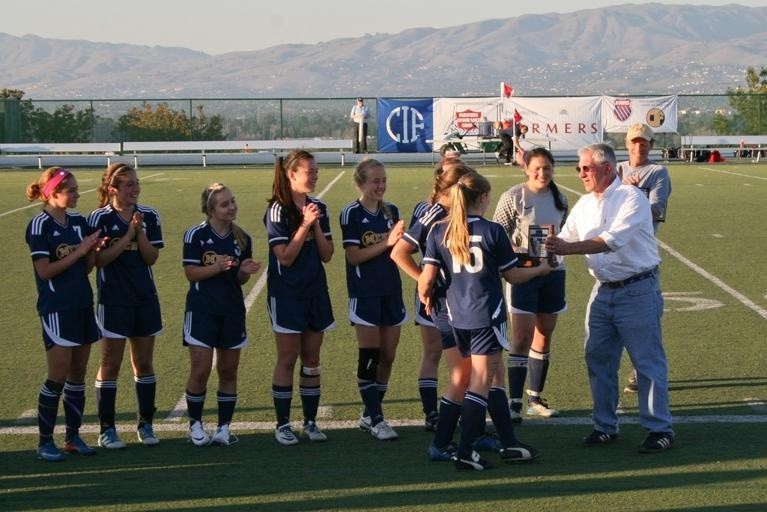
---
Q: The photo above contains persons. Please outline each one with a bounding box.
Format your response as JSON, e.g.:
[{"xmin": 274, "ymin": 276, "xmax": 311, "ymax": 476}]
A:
[
  {"xmin": 410, "ymin": 157, "xmax": 487, "ymax": 430},
  {"xmin": 498, "ymin": 124, "xmax": 527, "ymax": 167},
  {"xmin": 341, "ymin": 162, "xmax": 405, "ymax": 440},
  {"xmin": 27, "ymin": 165, "xmax": 93, "ymax": 461},
  {"xmin": 265, "ymin": 151, "xmax": 333, "ymax": 445},
  {"xmin": 612, "ymin": 122, "xmax": 670, "ymax": 392},
  {"xmin": 391, "ymin": 167, "xmax": 507, "ymax": 460},
  {"xmin": 419, "ymin": 174, "xmax": 554, "ymax": 471},
  {"xmin": 91, "ymin": 163, "xmax": 164, "ymax": 449},
  {"xmin": 544, "ymin": 144, "xmax": 674, "ymax": 451},
  {"xmin": 349, "ymin": 98, "xmax": 370, "ymax": 152},
  {"xmin": 493, "ymin": 149, "xmax": 567, "ymax": 421},
  {"xmin": 184, "ymin": 183, "xmax": 259, "ymax": 446}
]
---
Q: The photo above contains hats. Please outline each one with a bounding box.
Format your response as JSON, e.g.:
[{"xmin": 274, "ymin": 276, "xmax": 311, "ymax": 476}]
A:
[
  {"xmin": 356, "ymin": 96, "xmax": 365, "ymax": 102},
  {"xmin": 626, "ymin": 123, "xmax": 656, "ymax": 143}
]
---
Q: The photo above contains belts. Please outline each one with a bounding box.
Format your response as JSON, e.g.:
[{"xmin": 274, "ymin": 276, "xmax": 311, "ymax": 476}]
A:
[{"xmin": 600, "ymin": 267, "xmax": 659, "ymax": 288}]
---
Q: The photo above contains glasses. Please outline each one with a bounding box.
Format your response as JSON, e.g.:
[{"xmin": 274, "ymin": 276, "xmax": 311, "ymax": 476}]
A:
[{"xmin": 575, "ymin": 164, "xmax": 590, "ymax": 175}]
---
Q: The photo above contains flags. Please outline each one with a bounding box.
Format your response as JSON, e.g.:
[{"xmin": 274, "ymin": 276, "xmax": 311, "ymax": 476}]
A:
[
  {"xmin": 512, "ymin": 109, "xmax": 523, "ymax": 122},
  {"xmin": 503, "ymin": 83, "xmax": 514, "ymax": 99}
]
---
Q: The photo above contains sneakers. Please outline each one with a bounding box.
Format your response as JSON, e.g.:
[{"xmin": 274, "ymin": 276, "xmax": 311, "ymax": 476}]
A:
[
  {"xmin": 426, "ymin": 442, "xmax": 461, "ymax": 461},
  {"xmin": 509, "ymin": 407, "xmax": 524, "ymax": 423},
  {"xmin": 623, "ymin": 384, "xmax": 639, "ymax": 393},
  {"xmin": 211, "ymin": 425, "xmax": 232, "ymax": 447},
  {"xmin": 369, "ymin": 420, "xmax": 399, "ymax": 441},
  {"xmin": 494, "ymin": 150, "xmax": 500, "ymax": 159},
  {"xmin": 500, "ymin": 444, "xmax": 542, "ymax": 464},
  {"xmin": 38, "ymin": 440, "xmax": 61, "ymax": 463},
  {"xmin": 299, "ymin": 425, "xmax": 328, "ymax": 441},
  {"xmin": 275, "ymin": 420, "xmax": 299, "ymax": 446},
  {"xmin": 136, "ymin": 422, "xmax": 160, "ymax": 447},
  {"xmin": 583, "ymin": 431, "xmax": 619, "ymax": 447},
  {"xmin": 641, "ymin": 431, "xmax": 674, "ymax": 454},
  {"xmin": 504, "ymin": 162, "xmax": 512, "ymax": 164},
  {"xmin": 98, "ymin": 429, "xmax": 128, "ymax": 450},
  {"xmin": 188, "ymin": 422, "xmax": 211, "ymax": 447},
  {"xmin": 358, "ymin": 412, "xmax": 374, "ymax": 432},
  {"xmin": 527, "ymin": 401, "xmax": 560, "ymax": 420},
  {"xmin": 424, "ymin": 413, "xmax": 440, "ymax": 433},
  {"xmin": 471, "ymin": 437, "xmax": 507, "ymax": 454},
  {"xmin": 63, "ymin": 436, "xmax": 98, "ymax": 458},
  {"xmin": 452, "ymin": 450, "xmax": 492, "ymax": 472}
]
